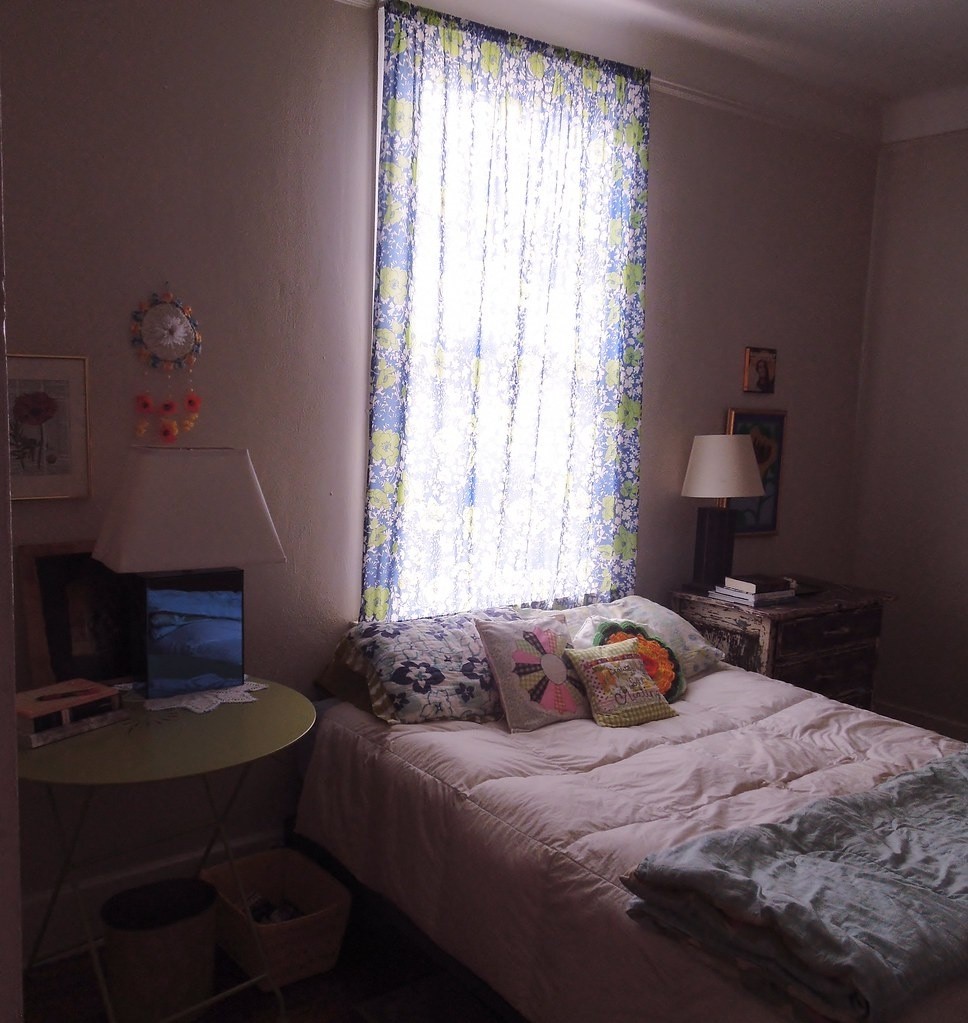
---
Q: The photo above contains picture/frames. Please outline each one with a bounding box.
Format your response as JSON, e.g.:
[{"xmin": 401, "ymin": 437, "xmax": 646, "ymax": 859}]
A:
[
  {"xmin": 717, "ymin": 408, "xmax": 788, "ymax": 538},
  {"xmin": 743, "ymin": 346, "xmax": 778, "ymax": 394},
  {"xmin": 4, "ymin": 352, "xmax": 92, "ymax": 501},
  {"xmin": 18, "ymin": 541, "xmax": 133, "ymax": 687}
]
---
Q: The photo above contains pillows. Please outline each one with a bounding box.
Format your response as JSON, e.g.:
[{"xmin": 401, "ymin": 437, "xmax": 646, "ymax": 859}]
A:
[
  {"xmin": 515, "ymin": 595, "xmax": 725, "ymax": 679},
  {"xmin": 564, "ymin": 638, "xmax": 679, "ymax": 727},
  {"xmin": 593, "ymin": 620, "xmax": 687, "ymax": 703},
  {"xmin": 473, "ymin": 614, "xmax": 593, "ymax": 734},
  {"xmin": 335, "ymin": 605, "xmax": 522, "ymax": 724}
]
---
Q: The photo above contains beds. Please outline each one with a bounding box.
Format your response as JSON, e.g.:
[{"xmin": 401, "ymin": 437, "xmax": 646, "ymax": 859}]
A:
[{"xmin": 290, "ymin": 598, "xmax": 968, "ymax": 1023}]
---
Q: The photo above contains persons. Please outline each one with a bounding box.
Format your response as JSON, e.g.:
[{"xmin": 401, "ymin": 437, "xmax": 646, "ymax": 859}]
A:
[{"xmin": 756, "ymin": 360, "xmax": 775, "ymax": 392}]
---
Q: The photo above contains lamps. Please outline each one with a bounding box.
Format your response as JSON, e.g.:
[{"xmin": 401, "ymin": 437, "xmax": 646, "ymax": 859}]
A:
[{"xmin": 680, "ymin": 434, "xmax": 767, "ymax": 587}]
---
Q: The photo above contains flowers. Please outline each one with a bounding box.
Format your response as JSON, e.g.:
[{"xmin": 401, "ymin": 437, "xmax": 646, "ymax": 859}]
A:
[{"xmin": 126, "ymin": 291, "xmax": 206, "ymax": 446}]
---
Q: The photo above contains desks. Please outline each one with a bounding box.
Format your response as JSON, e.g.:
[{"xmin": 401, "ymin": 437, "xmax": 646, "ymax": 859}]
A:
[{"xmin": 17, "ymin": 676, "xmax": 317, "ymax": 1023}]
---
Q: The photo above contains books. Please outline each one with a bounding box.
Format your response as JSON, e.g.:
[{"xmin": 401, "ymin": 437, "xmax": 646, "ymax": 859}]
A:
[
  {"xmin": 16, "ymin": 677, "xmax": 131, "ymax": 748},
  {"xmin": 708, "ymin": 573, "xmax": 798, "ymax": 607}
]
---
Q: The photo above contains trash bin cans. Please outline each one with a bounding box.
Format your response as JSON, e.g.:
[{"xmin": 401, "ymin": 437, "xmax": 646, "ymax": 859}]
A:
[{"xmin": 101, "ymin": 879, "xmax": 216, "ymax": 1023}]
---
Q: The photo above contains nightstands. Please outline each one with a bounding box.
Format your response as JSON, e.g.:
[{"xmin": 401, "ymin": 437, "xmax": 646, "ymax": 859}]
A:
[{"xmin": 668, "ymin": 572, "xmax": 896, "ymax": 709}]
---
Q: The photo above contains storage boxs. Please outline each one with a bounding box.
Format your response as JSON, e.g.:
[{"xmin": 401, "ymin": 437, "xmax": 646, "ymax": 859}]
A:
[{"xmin": 196, "ymin": 848, "xmax": 350, "ymax": 992}]
